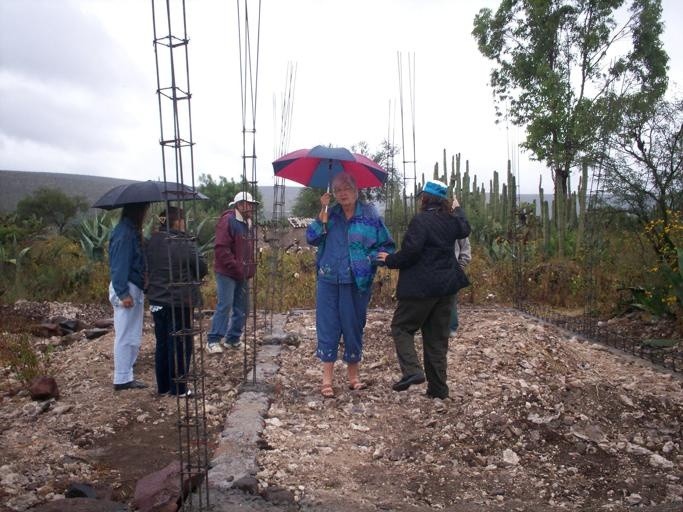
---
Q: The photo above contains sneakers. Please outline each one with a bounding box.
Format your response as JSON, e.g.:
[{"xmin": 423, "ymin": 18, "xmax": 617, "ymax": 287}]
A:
[
  {"xmin": 114, "ymin": 380, "xmax": 148, "ymax": 390},
  {"xmin": 204, "ymin": 341, "xmax": 223, "ymax": 355},
  {"xmin": 420, "ymin": 389, "xmax": 449, "ymax": 400},
  {"xmin": 223, "ymin": 341, "xmax": 250, "ymax": 350},
  {"xmin": 392, "ymin": 372, "xmax": 425, "ymax": 391}
]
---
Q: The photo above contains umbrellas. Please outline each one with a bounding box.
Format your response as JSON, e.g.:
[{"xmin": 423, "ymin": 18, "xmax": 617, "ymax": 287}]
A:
[{"xmin": 93, "ymin": 180, "xmax": 210, "ymax": 233}]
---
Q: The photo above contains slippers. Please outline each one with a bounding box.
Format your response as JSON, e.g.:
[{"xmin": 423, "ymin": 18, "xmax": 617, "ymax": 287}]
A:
[
  {"xmin": 348, "ymin": 382, "xmax": 367, "ymax": 390},
  {"xmin": 319, "ymin": 383, "xmax": 335, "ymax": 398}
]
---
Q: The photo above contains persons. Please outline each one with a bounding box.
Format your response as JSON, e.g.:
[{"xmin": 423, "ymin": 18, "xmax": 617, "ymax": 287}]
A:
[
  {"xmin": 448, "ymin": 236, "xmax": 472, "ymax": 335},
  {"xmin": 109, "ymin": 202, "xmax": 151, "ymax": 390},
  {"xmin": 305, "ymin": 171, "xmax": 397, "ymax": 397},
  {"xmin": 376, "ymin": 179, "xmax": 472, "ymax": 399},
  {"xmin": 145, "ymin": 207, "xmax": 209, "ymax": 399},
  {"xmin": 271, "ymin": 142, "xmax": 389, "ymax": 232},
  {"xmin": 203, "ymin": 191, "xmax": 259, "ymax": 354}
]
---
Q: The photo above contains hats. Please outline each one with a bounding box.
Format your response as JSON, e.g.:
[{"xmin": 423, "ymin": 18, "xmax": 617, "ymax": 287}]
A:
[
  {"xmin": 415, "ymin": 179, "xmax": 448, "ymax": 199},
  {"xmin": 228, "ymin": 191, "xmax": 259, "ymax": 207}
]
